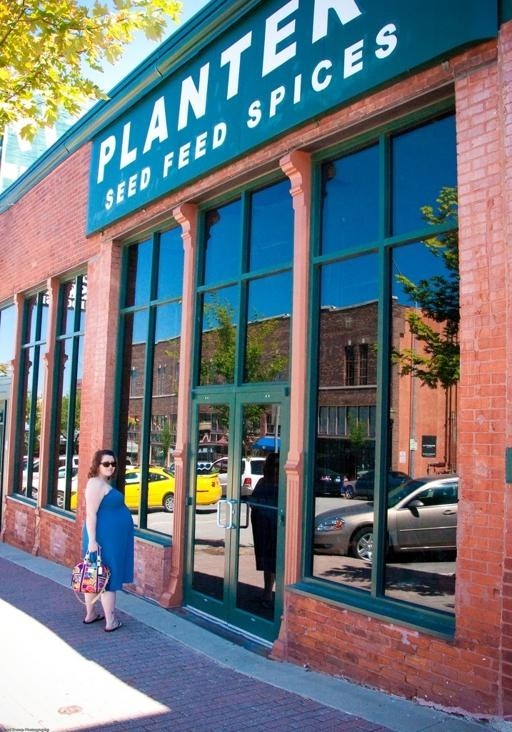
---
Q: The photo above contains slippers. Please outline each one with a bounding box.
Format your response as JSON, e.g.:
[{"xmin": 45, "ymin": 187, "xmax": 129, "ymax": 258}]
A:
[
  {"xmin": 105, "ymin": 621, "xmax": 123, "ymax": 632},
  {"xmin": 83, "ymin": 614, "xmax": 105, "ymax": 624}
]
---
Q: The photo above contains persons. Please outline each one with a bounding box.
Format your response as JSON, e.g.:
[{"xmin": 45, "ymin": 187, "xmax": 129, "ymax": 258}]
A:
[
  {"xmin": 247, "ymin": 452, "xmax": 280, "ymax": 607},
  {"xmin": 80, "ymin": 449, "xmax": 136, "ymax": 632}
]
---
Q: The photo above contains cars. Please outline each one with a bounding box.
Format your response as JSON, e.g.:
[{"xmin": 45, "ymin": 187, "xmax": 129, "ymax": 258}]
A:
[
  {"xmin": 23, "ymin": 452, "xmax": 220, "ymax": 517},
  {"xmin": 310, "ymin": 475, "xmax": 459, "ymax": 561},
  {"xmin": 315, "ymin": 465, "xmax": 341, "ymax": 495},
  {"xmin": 197, "ymin": 456, "xmax": 266, "ymax": 497},
  {"xmin": 339, "ymin": 470, "xmax": 409, "ymax": 499}
]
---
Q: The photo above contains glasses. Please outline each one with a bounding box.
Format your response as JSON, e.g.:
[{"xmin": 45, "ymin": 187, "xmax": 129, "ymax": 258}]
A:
[{"xmin": 100, "ymin": 462, "xmax": 116, "ymax": 468}]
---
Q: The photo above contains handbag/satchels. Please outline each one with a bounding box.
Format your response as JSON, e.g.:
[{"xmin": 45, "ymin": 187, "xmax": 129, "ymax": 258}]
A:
[{"xmin": 71, "ymin": 546, "xmax": 112, "ymax": 606}]
